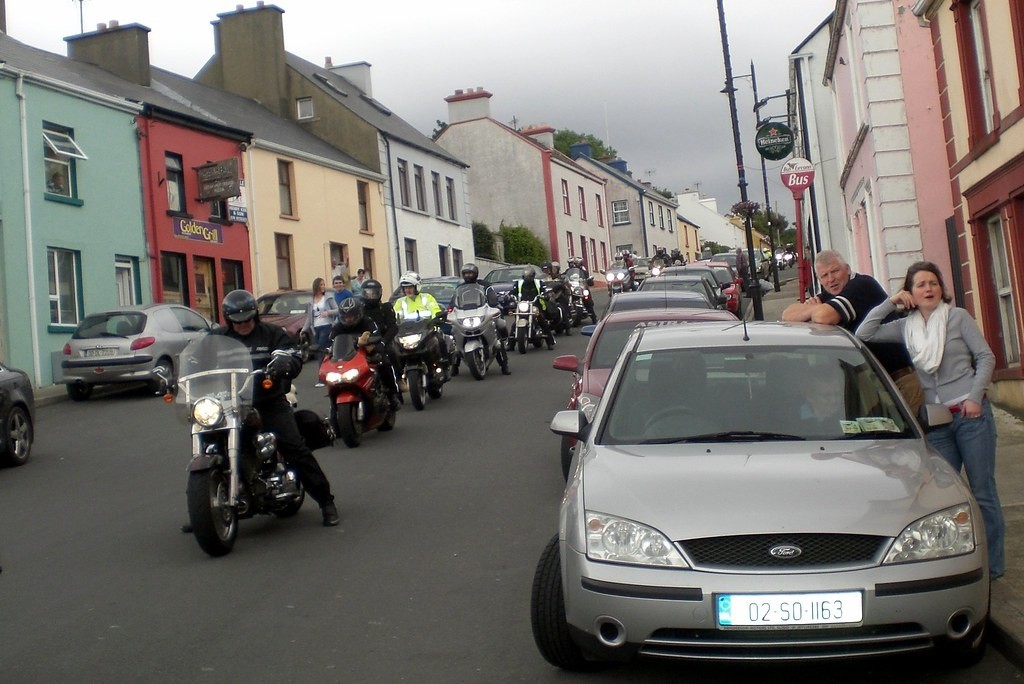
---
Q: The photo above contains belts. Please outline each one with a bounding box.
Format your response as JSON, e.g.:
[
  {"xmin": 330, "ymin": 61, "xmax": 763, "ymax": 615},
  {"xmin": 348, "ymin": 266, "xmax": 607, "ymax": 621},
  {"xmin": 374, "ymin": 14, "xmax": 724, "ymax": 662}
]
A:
[
  {"xmin": 891, "ymin": 366, "xmax": 916, "ymax": 381},
  {"xmin": 950, "ymin": 393, "xmax": 986, "ymax": 413}
]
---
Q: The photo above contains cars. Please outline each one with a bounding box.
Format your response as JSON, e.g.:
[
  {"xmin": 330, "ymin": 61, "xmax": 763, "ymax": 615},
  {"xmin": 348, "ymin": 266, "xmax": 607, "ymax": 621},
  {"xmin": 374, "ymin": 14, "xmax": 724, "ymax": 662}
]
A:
[
  {"xmin": 0, "ymin": 359, "xmax": 36, "ymax": 469},
  {"xmin": 581, "ymin": 248, "xmax": 771, "ymax": 336},
  {"xmin": 244, "ymin": 288, "xmax": 336, "ymax": 363},
  {"xmin": 389, "ymin": 276, "xmax": 464, "ymax": 317},
  {"xmin": 60, "ymin": 303, "xmax": 223, "ymax": 402},
  {"xmin": 530, "ymin": 320, "xmax": 992, "ymax": 675},
  {"xmin": 483, "ymin": 264, "xmax": 549, "ymax": 297},
  {"xmin": 552, "ymin": 308, "xmax": 742, "ymax": 484}
]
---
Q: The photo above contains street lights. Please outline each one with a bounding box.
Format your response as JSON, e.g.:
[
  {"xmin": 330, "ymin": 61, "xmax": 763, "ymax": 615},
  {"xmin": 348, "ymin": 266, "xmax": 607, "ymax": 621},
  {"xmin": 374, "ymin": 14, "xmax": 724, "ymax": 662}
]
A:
[{"xmin": 718, "ymin": 72, "xmax": 782, "ymax": 292}]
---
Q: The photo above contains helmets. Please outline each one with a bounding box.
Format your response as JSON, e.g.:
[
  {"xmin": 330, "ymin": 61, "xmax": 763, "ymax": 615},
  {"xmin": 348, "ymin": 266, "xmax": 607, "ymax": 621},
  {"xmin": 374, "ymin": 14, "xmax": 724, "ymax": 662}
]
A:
[
  {"xmin": 622, "ymin": 250, "xmax": 630, "ymax": 258},
  {"xmin": 400, "ymin": 271, "xmax": 422, "ymax": 295},
  {"xmin": 614, "ymin": 252, "xmax": 623, "ymax": 263},
  {"xmin": 551, "ymin": 261, "xmax": 560, "ymax": 274},
  {"xmin": 522, "ymin": 268, "xmax": 535, "ymax": 283},
  {"xmin": 361, "ymin": 280, "xmax": 383, "ymax": 307},
  {"xmin": 657, "ymin": 247, "xmax": 664, "ymax": 256},
  {"xmin": 461, "ymin": 263, "xmax": 478, "ymax": 283},
  {"xmin": 567, "ymin": 257, "xmax": 584, "ymax": 267},
  {"xmin": 671, "ymin": 247, "xmax": 681, "ymax": 258},
  {"xmin": 339, "ymin": 297, "xmax": 363, "ymax": 328},
  {"xmin": 222, "ymin": 290, "xmax": 261, "ymax": 327},
  {"xmin": 541, "ymin": 262, "xmax": 552, "ymax": 272}
]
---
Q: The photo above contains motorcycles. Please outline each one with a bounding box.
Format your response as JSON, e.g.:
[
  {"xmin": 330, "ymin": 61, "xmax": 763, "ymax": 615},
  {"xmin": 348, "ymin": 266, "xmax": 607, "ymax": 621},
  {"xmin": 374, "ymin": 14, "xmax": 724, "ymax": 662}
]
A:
[
  {"xmin": 152, "ymin": 333, "xmax": 336, "ymax": 558},
  {"xmin": 498, "ymin": 286, "xmax": 558, "ymax": 355},
  {"xmin": 768, "ymin": 249, "xmax": 799, "ymax": 274},
  {"xmin": 305, "ymin": 334, "xmax": 396, "ymax": 447},
  {"xmin": 442, "ymin": 283, "xmax": 509, "ymax": 379},
  {"xmin": 544, "ymin": 267, "xmax": 595, "ymax": 336},
  {"xmin": 393, "ymin": 312, "xmax": 450, "ymax": 411},
  {"xmin": 599, "ymin": 267, "xmax": 636, "ymax": 297}
]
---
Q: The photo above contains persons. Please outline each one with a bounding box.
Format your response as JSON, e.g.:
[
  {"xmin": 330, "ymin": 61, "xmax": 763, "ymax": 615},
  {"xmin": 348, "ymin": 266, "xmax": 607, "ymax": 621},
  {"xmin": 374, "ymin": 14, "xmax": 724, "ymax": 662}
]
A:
[
  {"xmin": 177, "ymin": 288, "xmax": 340, "ymax": 532},
  {"xmin": 763, "ymin": 240, "xmax": 797, "ymax": 260},
  {"xmin": 735, "ymin": 247, "xmax": 752, "ymax": 298},
  {"xmin": 780, "ymin": 363, "xmax": 849, "ymax": 428},
  {"xmin": 854, "ymin": 261, "xmax": 1004, "ymax": 582},
  {"xmin": 299, "ymin": 278, "xmax": 339, "ymax": 388},
  {"xmin": 320, "ymin": 247, "xmax": 686, "ymax": 424},
  {"xmin": 782, "ymin": 249, "xmax": 925, "ymax": 421}
]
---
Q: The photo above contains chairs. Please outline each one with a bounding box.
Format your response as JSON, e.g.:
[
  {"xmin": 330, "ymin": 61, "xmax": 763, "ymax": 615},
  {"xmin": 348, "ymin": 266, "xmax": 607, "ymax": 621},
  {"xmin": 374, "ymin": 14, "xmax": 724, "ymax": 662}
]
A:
[{"xmin": 116, "ymin": 322, "xmax": 134, "ymax": 336}]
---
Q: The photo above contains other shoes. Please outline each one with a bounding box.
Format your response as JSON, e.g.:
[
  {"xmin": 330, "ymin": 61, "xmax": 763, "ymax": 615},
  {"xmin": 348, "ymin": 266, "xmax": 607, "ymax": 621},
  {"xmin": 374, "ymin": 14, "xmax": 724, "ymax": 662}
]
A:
[
  {"xmin": 322, "ymin": 503, "xmax": 339, "ymax": 524},
  {"xmin": 566, "ymin": 327, "xmax": 572, "ymax": 336},
  {"xmin": 315, "ymin": 383, "xmax": 325, "ymax": 387},
  {"xmin": 180, "ymin": 524, "xmax": 193, "ymax": 532},
  {"xmin": 548, "ymin": 343, "xmax": 553, "ymax": 350},
  {"xmin": 390, "ymin": 397, "xmax": 400, "ymax": 410},
  {"xmin": 450, "ymin": 366, "xmax": 459, "ymax": 377},
  {"xmin": 506, "ymin": 345, "xmax": 515, "ymax": 351},
  {"xmin": 502, "ymin": 366, "xmax": 512, "ymax": 375}
]
